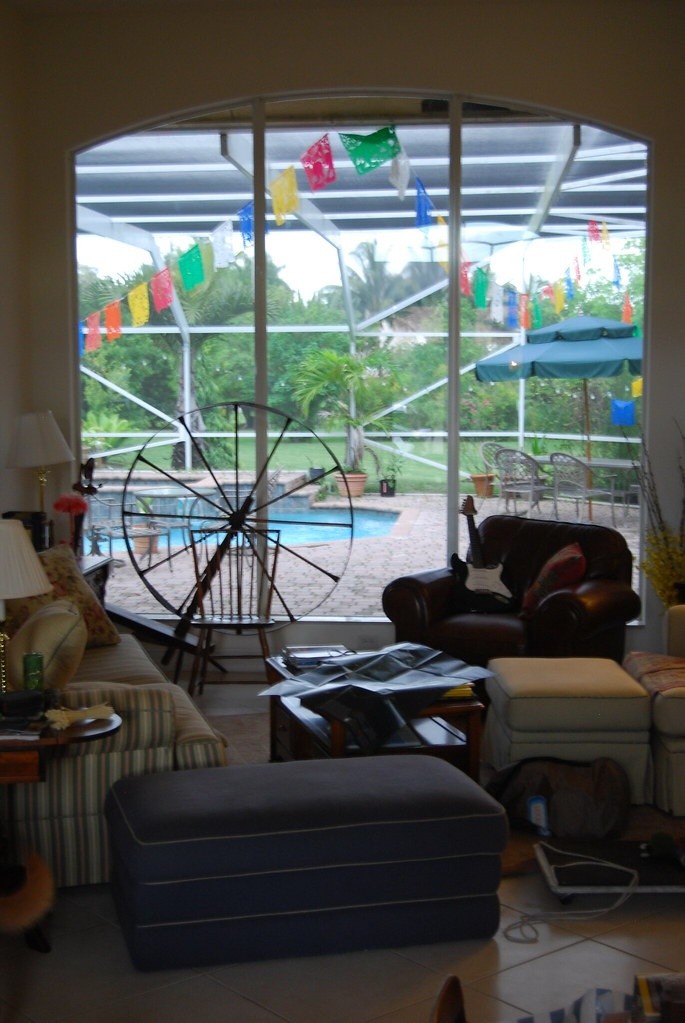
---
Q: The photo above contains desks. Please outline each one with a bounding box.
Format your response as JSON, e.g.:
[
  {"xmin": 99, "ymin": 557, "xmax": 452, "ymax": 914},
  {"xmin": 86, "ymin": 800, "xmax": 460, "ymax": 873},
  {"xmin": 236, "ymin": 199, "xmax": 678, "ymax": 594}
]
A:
[
  {"xmin": 133, "ymin": 488, "xmax": 216, "ymax": 560},
  {"xmin": 506, "ymin": 456, "xmax": 641, "ymax": 470}
]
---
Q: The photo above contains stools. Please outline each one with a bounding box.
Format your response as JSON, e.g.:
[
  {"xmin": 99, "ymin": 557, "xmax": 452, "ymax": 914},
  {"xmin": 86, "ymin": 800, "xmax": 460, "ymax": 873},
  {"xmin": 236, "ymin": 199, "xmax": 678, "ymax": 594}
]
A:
[{"xmin": 486, "ymin": 658, "xmax": 652, "ymax": 806}]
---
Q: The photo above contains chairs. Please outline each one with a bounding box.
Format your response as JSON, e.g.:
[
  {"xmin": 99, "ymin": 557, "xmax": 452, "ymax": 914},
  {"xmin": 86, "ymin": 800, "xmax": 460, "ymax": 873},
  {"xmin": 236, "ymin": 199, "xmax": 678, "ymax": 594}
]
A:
[
  {"xmin": 476, "ymin": 442, "xmax": 641, "ymax": 528},
  {"xmin": 84, "ymin": 466, "xmax": 285, "ymax": 694}
]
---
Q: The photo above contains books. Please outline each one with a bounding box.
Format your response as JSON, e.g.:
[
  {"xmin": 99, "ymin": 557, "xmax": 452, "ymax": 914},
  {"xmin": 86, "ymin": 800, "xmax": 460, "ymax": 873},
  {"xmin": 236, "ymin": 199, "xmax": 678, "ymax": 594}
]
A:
[
  {"xmin": 0, "ymin": 720, "xmax": 48, "ymax": 741},
  {"xmin": 281, "ymin": 644, "xmax": 348, "ymax": 669}
]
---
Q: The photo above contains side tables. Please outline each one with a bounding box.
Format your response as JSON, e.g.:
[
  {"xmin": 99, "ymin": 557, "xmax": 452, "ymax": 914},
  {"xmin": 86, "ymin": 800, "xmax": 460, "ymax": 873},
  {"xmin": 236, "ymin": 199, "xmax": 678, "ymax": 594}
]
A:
[{"xmin": 0, "ymin": 708, "xmax": 123, "ymax": 954}]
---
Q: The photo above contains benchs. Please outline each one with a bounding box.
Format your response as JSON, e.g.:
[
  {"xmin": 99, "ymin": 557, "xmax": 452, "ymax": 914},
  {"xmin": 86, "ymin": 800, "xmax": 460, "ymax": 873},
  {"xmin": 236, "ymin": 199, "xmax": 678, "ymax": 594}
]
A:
[{"xmin": 100, "ymin": 755, "xmax": 509, "ymax": 976}]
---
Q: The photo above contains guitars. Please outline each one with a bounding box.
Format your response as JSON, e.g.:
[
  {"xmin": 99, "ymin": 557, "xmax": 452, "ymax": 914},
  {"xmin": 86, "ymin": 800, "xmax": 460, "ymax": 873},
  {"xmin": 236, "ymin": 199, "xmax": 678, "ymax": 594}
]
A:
[{"xmin": 446, "ymin": 495, "xmax": 524, "ymax": 615}]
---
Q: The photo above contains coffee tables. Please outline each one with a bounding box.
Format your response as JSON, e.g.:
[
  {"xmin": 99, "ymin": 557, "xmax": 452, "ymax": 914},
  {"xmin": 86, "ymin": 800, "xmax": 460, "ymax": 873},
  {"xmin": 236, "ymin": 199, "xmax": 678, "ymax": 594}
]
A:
[{"xmin": 264, "ymin": 657, "xmax": 485, "ymax": 784}]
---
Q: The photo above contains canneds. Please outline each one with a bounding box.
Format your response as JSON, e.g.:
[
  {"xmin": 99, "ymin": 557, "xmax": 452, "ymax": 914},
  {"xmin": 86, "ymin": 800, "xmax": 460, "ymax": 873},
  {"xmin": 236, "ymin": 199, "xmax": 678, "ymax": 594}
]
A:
[
  {"xmin": 23, "ymin": 651, "xmax": 45, "ymax": 690},
  {"xmin": 527, "ymin": 795, "xmax": 551, "ymax": 836}
]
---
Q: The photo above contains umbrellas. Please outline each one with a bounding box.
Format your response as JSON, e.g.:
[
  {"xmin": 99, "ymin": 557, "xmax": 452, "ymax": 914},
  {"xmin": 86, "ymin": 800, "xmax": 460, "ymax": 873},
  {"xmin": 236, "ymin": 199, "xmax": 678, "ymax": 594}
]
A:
[{"xmin": 472, "ymin": 317, "xmax": 644, "ymax": 518}]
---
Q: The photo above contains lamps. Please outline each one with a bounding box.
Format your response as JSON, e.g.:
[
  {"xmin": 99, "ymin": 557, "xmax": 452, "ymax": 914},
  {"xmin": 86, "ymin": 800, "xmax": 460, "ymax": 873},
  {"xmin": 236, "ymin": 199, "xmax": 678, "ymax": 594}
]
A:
[
  {"xmin": 5, "ymin": 409, "xmax": 75, "ymax": 512},
  {"xmin": 0, "ymin": 519, "xmax": 55, "ymax": 602}
]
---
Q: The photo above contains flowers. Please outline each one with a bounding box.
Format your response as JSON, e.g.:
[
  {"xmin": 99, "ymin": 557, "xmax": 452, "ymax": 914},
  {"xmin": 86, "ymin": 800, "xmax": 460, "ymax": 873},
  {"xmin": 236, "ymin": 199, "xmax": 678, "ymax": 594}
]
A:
[{"xmin": 631, "ymin": 521, "xmax": 685, "ymax": 608}]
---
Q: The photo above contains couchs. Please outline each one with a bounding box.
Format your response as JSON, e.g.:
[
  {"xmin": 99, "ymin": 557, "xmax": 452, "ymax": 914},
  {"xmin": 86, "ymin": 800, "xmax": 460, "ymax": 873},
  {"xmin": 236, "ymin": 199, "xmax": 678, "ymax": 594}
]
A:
[
  {"xmin": 381, "ymin": 514, "xmax": 642, "ymax": 723},
  {"xmin": 0, "ymin": 634, "xmax": 230, "ymax": 887},
  {"xmin": 623, "ymin": 605, "xmax": 685, "ymax": 815}
]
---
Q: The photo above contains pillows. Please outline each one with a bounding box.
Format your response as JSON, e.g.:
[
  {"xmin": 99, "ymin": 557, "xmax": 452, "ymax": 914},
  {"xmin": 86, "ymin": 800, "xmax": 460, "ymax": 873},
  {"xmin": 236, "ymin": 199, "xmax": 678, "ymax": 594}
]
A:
[
  {"xmin": 4, "ymin": 598, "xmax": 88, "ymax": 691},
  {"xmin": 2, "ymin": 542, "xmax": 121, "ymax": 645},
  {"xmin": 521, "ymin": 542, "xmax": 585, "ymax": 607}
]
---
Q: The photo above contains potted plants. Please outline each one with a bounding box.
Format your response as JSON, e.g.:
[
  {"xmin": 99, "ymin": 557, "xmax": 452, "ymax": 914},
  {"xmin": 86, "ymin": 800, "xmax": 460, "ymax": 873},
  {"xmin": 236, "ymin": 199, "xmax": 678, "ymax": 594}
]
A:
[
  {"xmin": 470, "ymin": 464, "xmax": 495, "ymax": 496},
  {"xmin": 333, "ymin": 464, "xmax": 367, "ymax": 497},
  {"xmin": 379, "ymin": 449, "xmax": 407, "ymax": 497}
]
